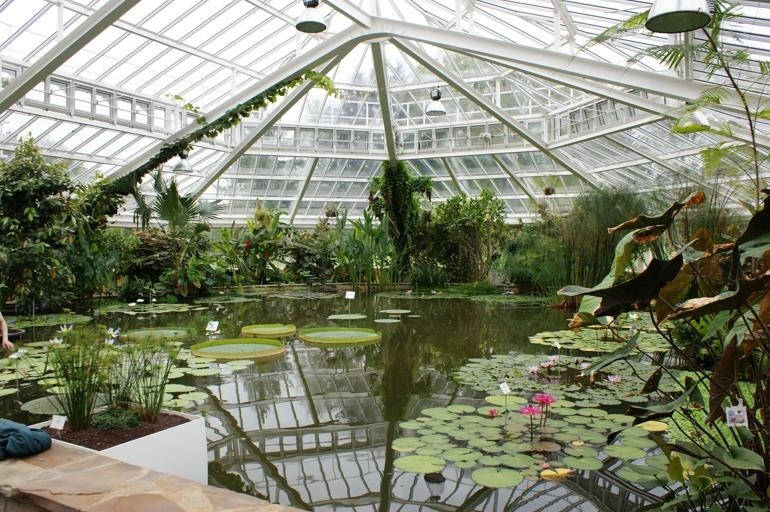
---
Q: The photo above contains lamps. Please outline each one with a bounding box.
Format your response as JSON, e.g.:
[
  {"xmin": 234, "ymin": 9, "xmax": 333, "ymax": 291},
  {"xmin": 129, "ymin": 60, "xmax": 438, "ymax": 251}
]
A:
[
  {"xmin": 645, "ymin": 0, "xmax": 712, "ymax": 34},
  {"xmin": 296, "ymin": 0, "xmax": 326, "ymax": 34},
  {"xmin": 426, "ymin": 87, "xmax": 447, "ymax": 116}
]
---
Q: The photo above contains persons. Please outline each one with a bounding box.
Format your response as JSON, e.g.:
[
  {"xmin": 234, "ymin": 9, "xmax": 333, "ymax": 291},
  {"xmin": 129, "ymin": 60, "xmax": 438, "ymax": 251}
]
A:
[{"xmin": 0, "ymin": 309, "xmax": 15, "ymax": 352}]
